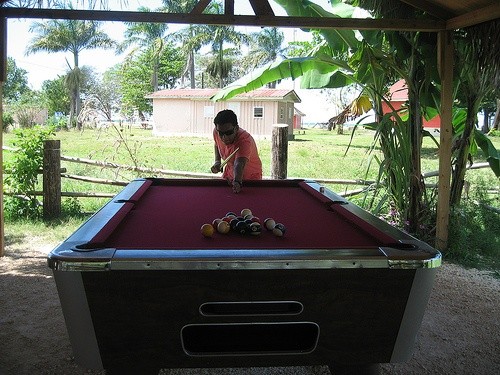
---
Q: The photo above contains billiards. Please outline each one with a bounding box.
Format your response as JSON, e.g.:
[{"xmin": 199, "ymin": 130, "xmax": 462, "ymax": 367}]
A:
[
  {"xmin": 227, "ymin": 215, "xmax": 236, "ymax": 219},
  {"xmin": 235, "ymin": 216, "xmax": 244, "ymax": 221},
  {"xmin": 244, "ymin": 215, "xmax": 254, "ymax": 220},
  {"xmin": 241, "ymin": 208, "xmax": 252, "ymax": 216},
  {"xmin": 222, "ymin": 217, "xmax": 232, "ymax": 222},
  {"xmin": 211, "ymin": 218, "xmax": 222, "ymax": 228},
  {"xmin": 263, "ymin": 217, "xmax": 275, "ymax": 230},
  {"xmin": 230, "ymin": 219, "xmax": 239, "ymax": 230},
  {"xmin": 250, "ymin": 217, "xmax": 260, "ymax": 222},
  {"xmin": 236, "ymin": 222, "xmax": 248, "ymax": 233},
  {"xmin": 199, "ymin": 223, "xmax": 215, "ymax": 238},
  {"xmin": 217, "ymin": 221, "xmax": 230, "ymax": 234},
  {"xmin": 250, "ymin": 222, "xmax": 262, "ymax": 235},
  {"xmin": 273, "ymin": 223, "xmax": 287, "ymax": 237},
  {"xmin": 226, "ymin": 212, "xmax": 237, "ymax": 216},
  {"xmin": 245, "ymin": 219, "xmax": 253, "ymax": 225}
]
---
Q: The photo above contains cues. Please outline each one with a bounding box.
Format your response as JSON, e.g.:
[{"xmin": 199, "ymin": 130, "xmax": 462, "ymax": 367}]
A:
[{"xmin": 218, "ymin": 147, "xmax": 240, "ymax": 170}]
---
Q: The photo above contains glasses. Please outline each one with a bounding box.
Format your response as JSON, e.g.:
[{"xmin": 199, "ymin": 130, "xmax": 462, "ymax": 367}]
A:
[{"xmin": 215, "ymin": 124, "xmax": 237, "ymax": 136}]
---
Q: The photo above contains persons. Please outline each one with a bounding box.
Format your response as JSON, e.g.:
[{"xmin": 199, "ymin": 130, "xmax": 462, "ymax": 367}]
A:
[{"xmin": 210, "ymin": 108, "xmax": 262, "ymax": 193}]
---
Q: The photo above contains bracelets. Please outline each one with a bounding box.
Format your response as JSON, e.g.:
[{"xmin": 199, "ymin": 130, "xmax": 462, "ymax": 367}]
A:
[{"xmin": 233, "ymin": 178, "xmax": 242, "ymax": 185}]
